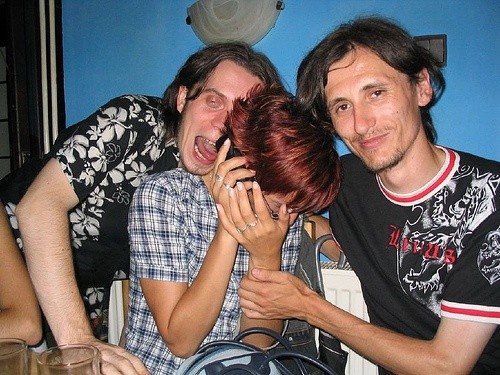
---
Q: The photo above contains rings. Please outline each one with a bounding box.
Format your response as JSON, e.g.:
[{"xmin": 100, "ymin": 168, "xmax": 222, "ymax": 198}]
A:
[
  {"xmin": 214, "ymin": 175, "xmax": 224, "ymax": 182},
  {"xmin": 239, "ymin": 226, "xmax": 247, "ymax": 232},
  {"xmin": 223, "ymin": 182, "xmax": 230, "ymax": 189},
  {"xmin": 247, "ymin": 220, "xmax": 257, "ymax": 228}
]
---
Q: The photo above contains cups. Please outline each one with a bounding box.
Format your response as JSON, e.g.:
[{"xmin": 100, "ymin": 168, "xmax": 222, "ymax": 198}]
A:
[
  {"xmin": 38, "ymin": 343, "xmax": 100, "ymax": 375},
  {"xmin": 0, "ymin": 338, "xmax": 28, "ymax": 375}
]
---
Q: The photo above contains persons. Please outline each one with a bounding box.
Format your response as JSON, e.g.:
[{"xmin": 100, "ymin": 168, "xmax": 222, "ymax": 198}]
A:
[
  {"xmin": 122, "ymin": 85, "xmax": 344, "ymax": 375},
  {"xmin": 0, "ymin": 41, "xmax": 287, "ymax": 375},
  {"xmin": 0, "ymin": 201, "xmax": 47, "ymax": 353},
  {"xmin": 239, "ymin": 16, "xmax": 500, "ymax": 375}
]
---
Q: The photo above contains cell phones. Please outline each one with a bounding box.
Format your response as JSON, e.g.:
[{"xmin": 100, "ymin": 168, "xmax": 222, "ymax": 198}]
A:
[{"xmin": 216, "ymin": 135, "xmax": 252, "ymax": 186}]
